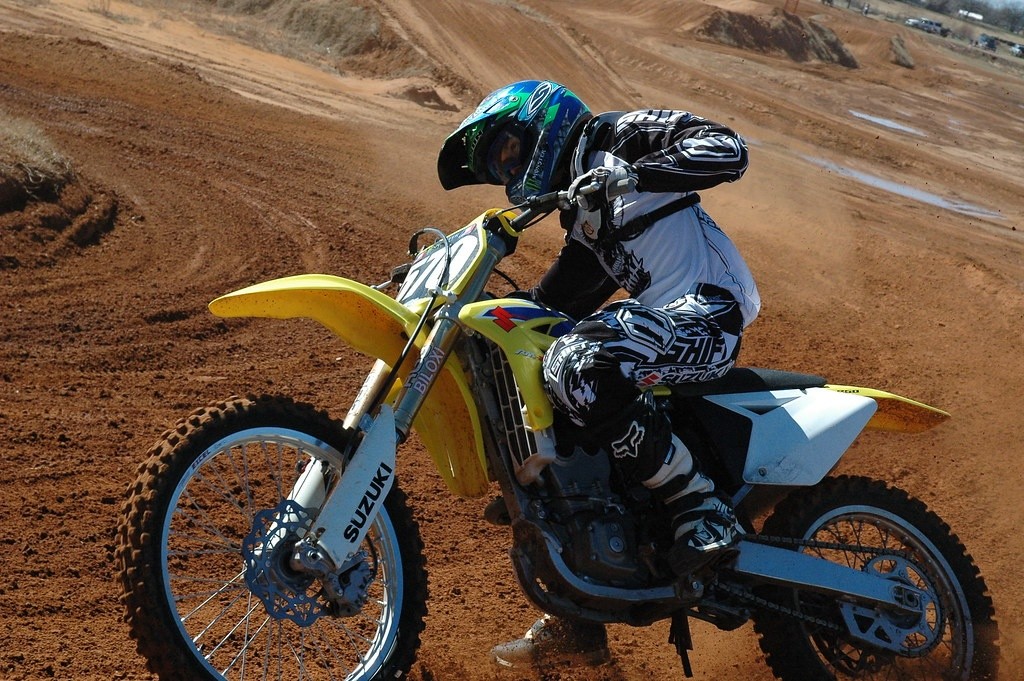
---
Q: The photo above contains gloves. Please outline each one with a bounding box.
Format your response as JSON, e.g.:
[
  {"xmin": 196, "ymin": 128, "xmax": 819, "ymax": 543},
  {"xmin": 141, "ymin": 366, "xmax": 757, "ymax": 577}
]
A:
[{"xmin": 581, "ymin": 151, "xmax": 636, "ymax": 212}]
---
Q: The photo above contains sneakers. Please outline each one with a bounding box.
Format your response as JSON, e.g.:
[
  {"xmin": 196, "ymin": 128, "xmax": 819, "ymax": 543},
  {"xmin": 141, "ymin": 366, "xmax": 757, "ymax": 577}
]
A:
[
  {"xmin": 669, "ymin": 496, "xmax": 744, "ymax": 577},
  {"xmin": 489, "ymin": 613, "xmax": 610, "ymax": 667}
]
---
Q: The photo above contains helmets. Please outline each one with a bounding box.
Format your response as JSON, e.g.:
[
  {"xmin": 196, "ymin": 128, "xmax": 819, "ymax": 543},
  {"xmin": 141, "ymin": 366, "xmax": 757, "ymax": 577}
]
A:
[{"xmin": 437, "ymin": 79, "xmax": 595, "ymax": 204}]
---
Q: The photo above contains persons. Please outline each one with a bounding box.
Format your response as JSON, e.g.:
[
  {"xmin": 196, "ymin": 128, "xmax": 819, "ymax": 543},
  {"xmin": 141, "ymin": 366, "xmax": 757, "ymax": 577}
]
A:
[{"xmin": 436, "ymin": 82, "xmax": 761, "ymax": 666}]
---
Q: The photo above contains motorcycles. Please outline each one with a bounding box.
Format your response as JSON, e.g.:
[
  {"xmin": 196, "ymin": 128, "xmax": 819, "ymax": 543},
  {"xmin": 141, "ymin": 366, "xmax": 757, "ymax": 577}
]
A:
[{"xmin": 113, "ymin": 181, "xmax": 1000, "ymax": 681}]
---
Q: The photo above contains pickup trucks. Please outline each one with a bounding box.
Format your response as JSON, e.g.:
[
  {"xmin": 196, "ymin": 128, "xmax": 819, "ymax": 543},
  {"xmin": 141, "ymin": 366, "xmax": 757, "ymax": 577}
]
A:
[
  {"xmin": 1012, "ymin": 45, "xmax": 1024, "ymax": 58},
  {"xmin": 906, "ymin": 19, "xmax": 942, "ymax": 34}
]
